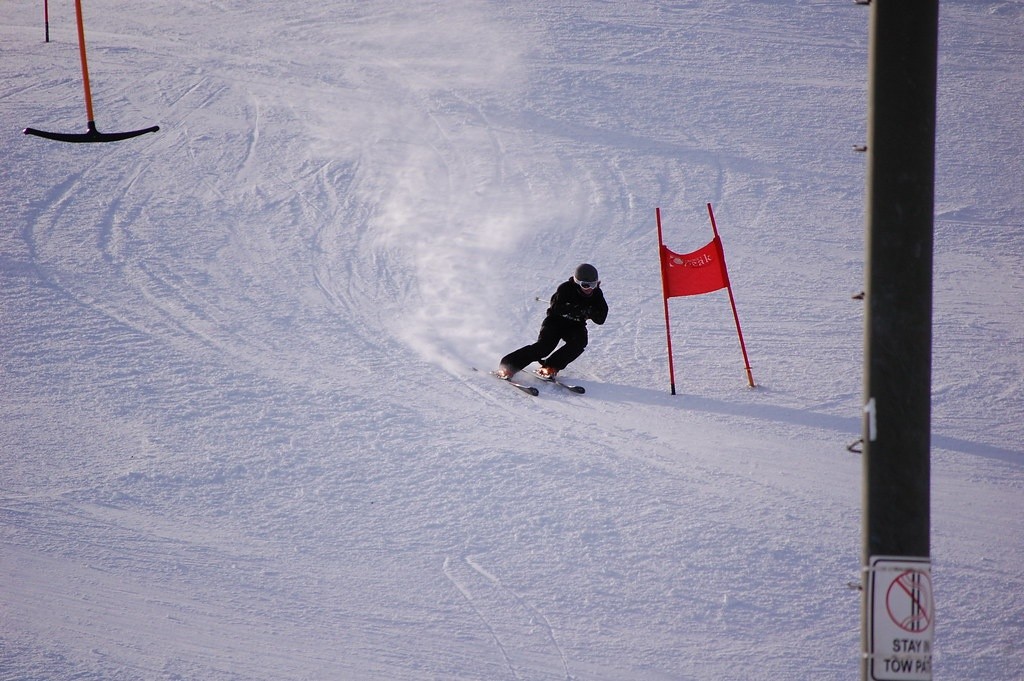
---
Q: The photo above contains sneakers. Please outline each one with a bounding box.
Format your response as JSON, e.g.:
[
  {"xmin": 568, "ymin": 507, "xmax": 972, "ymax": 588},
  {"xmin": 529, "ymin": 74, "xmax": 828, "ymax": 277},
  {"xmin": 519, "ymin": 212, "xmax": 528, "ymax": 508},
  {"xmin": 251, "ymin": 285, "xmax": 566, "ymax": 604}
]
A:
[
  {"xmin": 497, "ymin": 364, "xmax": 514, "ymax": 379},
  {"xmin": 538, "ymin": 366, "xmax": 558, "ymax": 379}
]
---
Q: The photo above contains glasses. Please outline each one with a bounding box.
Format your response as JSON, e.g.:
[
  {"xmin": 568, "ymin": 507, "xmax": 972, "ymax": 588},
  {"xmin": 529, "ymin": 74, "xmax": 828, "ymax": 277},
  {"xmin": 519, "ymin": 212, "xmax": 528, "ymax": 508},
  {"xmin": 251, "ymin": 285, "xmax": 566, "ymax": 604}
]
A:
[{"xmin": 574, "ymin": 276, "xmax": 598, "ymax": 290}]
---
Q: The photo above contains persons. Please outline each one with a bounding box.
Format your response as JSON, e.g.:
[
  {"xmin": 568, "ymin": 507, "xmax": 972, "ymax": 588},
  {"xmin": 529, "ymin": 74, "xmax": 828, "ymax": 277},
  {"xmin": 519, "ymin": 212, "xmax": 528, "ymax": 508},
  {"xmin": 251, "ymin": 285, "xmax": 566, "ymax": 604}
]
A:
[{"xmin": 497, "ymin": 263, "xmax": 609, "ymax": 381}]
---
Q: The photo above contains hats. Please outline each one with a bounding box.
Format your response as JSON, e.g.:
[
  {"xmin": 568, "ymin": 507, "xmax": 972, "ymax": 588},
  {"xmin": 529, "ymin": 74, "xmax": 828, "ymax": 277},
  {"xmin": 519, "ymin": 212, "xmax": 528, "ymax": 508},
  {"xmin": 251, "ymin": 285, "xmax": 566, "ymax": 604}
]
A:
[{"xmin": 575, "ymin": 264, "xmax": 598, "ymax": 282}]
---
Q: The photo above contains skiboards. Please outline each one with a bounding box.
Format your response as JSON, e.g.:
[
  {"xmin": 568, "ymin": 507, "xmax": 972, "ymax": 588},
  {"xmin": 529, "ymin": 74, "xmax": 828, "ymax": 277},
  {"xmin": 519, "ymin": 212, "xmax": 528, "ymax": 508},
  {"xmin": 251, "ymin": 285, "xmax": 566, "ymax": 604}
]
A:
[{"xmin": 471, "ymin": 360, "xmax": 586, "ymax": 396}]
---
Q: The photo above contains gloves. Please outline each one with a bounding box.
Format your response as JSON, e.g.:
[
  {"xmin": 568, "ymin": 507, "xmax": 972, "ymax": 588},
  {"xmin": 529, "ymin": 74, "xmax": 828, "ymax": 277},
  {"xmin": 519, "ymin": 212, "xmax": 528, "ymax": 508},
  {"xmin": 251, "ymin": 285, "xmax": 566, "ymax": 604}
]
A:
[
  {"xmin": 563, "ymin": 303, "xmax": 572, "ymax": 315},
  {"xmin": 587, "ymin": 306, "xmax": 597, "ymax": 318}
]
